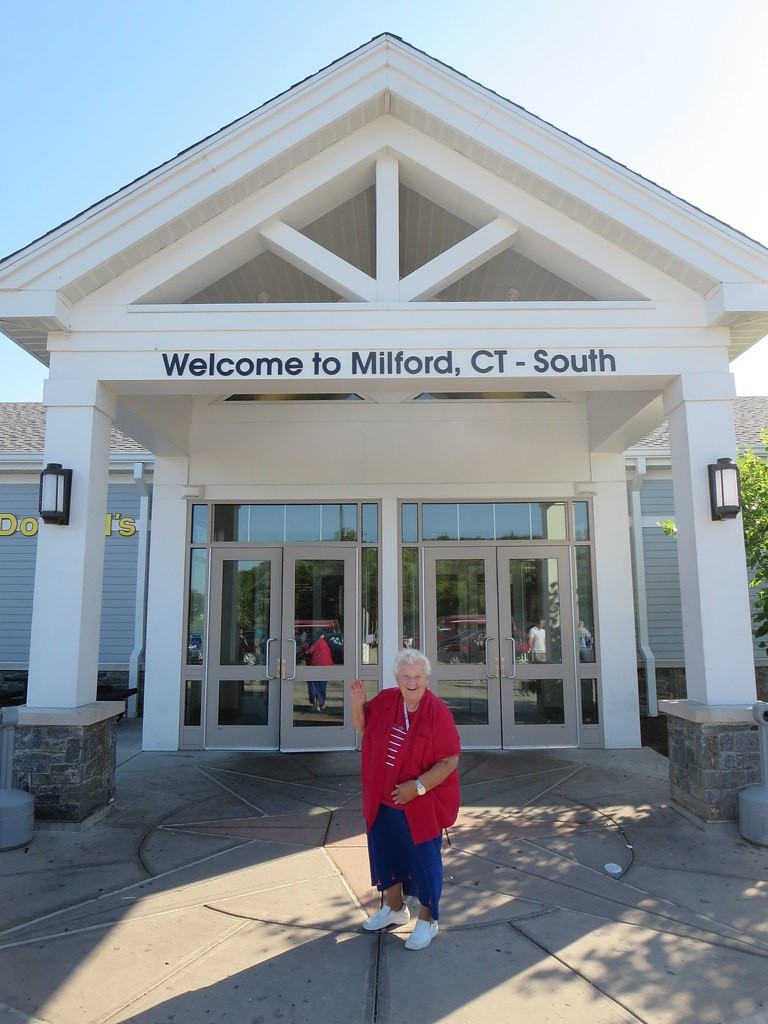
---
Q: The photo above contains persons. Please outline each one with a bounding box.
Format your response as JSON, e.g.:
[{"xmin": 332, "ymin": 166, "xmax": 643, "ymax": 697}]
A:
[
  {"xmin": 579, "ymin": 620, "xmax": 591, "ymax": 648},
  {"xmin": 304, "ymin": 628, "xmax": 333, "ymax": 711},
  {"xmin": 350, "ymin": 649, "xmax": 460, "ymax": 950},
  {"xmin": 528, "ymin": 619, "xmax": 548, "ymax": 662},
  {"xmin": 239, "ymin": 627, "xmax": 248, "ymax": 663}
]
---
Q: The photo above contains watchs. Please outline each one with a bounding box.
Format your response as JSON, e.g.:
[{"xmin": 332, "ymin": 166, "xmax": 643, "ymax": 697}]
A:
[{"xmin": 416, "ymin": 780, "xmax": 426, "ymax": 795}]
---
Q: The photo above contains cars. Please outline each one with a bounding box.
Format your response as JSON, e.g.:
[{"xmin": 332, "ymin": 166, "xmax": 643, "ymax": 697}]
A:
[
  {"xmin": 238, "ymin": 633, "xmax": 263, "ymax": 665},
  {"xmin": 439, "ymin": 630, "xmax": 536, "ymax": 666}
]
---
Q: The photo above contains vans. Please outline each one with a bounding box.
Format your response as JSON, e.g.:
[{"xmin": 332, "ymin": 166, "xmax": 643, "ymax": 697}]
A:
[
  {"xmin": 438, "ymin": 613, "xmax": 519, "ymax": 648},
  {"xmin": 294, "ymin": 616, "xmax": 341, "ymax": 636}
]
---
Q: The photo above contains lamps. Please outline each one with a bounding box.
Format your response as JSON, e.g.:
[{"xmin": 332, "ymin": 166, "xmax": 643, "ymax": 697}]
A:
[
  {"xmin": 707, "ymin": 458, "xmax": 741, "ymax": 522},
  {"xmin": 38, "ymin": 462, "xmax": 73, "ymax": 526}
]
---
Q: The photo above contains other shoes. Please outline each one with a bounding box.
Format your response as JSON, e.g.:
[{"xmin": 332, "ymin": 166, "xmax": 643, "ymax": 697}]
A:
[
  {"xmin": 363, "ymin": 904, "xmax": 410, "ymax": 930},
  {"xmin": 405, "ymin": 919, "xmax": 438, "ymax": 950},
  {"xmin": 320, "ymin": 705, "xmax": 325, "ymax": 709},
  {"xmin": 310, "ymin": 706, "xmax": 320, "ymax": 711}
]
